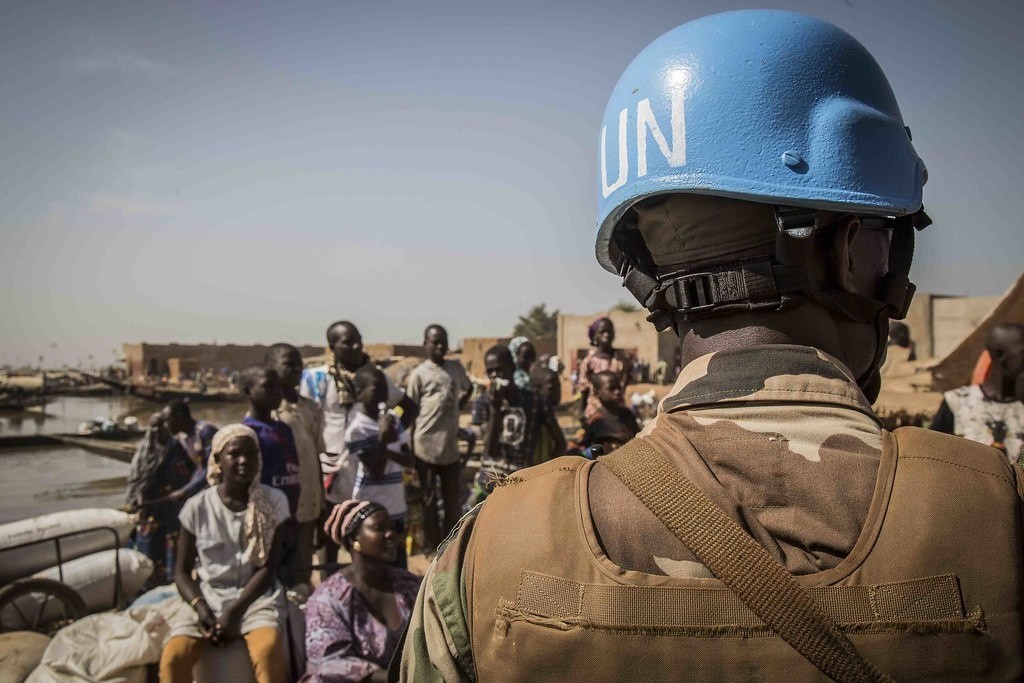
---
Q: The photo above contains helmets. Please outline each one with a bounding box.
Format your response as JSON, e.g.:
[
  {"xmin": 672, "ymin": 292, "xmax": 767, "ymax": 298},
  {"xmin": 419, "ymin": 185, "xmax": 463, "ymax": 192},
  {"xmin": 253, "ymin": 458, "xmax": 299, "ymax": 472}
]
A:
[{"xmin": 591, "ymin": 9, "xmax": 928, "ymax": 276}]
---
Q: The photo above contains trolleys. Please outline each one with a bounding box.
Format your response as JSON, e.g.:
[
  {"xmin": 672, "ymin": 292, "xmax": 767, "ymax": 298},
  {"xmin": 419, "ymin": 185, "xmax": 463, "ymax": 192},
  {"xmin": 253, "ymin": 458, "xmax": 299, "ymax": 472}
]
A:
[{"xmin": 0, "ymin": 496, "xmax": 174, "ymax": 638}]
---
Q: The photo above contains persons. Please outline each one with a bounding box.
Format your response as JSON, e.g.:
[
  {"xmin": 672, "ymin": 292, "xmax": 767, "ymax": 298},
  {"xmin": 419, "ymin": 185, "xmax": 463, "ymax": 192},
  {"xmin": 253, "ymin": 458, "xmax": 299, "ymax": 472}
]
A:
[
  {"xmin": 126, "ymin": 316, "xmax": 1024, "ymax": 683},
  {"xmin": 399, "ymin": 8, "xmax": 1024, "ymax": 683}
]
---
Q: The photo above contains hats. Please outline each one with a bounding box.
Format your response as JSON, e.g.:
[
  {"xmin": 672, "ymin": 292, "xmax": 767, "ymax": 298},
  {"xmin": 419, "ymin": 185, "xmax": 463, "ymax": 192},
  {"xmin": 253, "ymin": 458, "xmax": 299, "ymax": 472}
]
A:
[{"xmin": 324, "ymin": 498, "xmax": 387, "ymax": 550}]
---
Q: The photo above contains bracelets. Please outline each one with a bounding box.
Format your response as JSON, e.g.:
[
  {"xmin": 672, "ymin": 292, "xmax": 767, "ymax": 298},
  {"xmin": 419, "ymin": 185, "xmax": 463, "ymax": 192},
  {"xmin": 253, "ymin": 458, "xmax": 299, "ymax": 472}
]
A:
[{"xmin": 190, "ymin": 595, "xmax": 207, "ymax": 609}]
